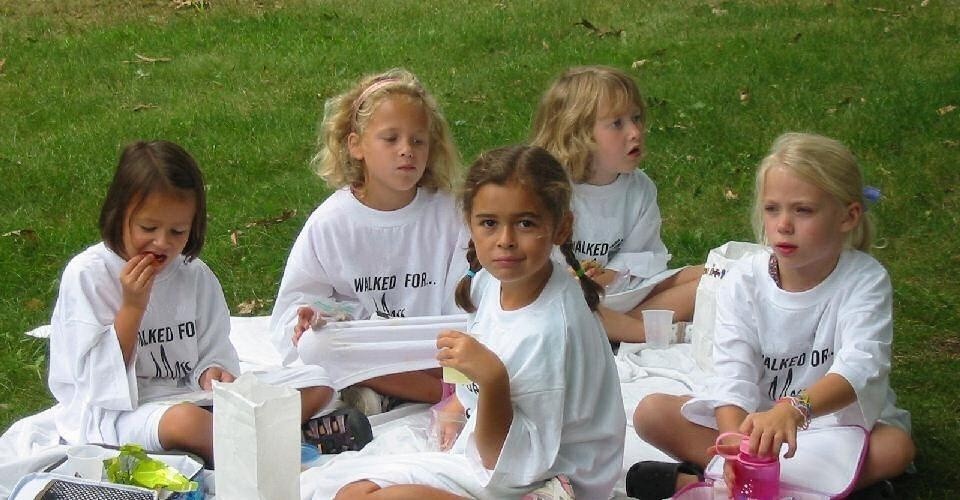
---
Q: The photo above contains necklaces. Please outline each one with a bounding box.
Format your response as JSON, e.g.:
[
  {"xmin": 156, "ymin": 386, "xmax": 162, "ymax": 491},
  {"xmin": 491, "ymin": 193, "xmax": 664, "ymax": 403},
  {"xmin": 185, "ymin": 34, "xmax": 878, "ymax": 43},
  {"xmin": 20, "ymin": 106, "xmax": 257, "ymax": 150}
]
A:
[{"xmin": 769, "ymin": 254, "xmax": 780, "ymax": 287}]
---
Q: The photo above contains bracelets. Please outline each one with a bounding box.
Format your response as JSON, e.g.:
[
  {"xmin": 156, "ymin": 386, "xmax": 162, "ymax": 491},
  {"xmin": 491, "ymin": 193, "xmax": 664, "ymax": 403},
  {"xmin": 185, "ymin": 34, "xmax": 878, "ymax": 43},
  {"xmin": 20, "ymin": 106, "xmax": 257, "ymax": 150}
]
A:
[{"xmin": 776, "ymin": 390, "xmax": 814, "ymax": 430}]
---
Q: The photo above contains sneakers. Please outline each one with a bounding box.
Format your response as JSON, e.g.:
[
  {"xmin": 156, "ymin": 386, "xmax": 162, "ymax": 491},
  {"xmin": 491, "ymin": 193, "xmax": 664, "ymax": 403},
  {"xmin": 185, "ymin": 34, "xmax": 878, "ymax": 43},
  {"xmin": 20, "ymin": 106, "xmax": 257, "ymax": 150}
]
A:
[
  {"xmin": 668, "ymin": 319, "xmax": 696, "ymax": 346},
  {"xmin": 521, "ymin": 472, "xmax": 576, "ymax": 500},
  {"xmin": 339, "ymin": 385, "xmax": 395, "ymax": 417}
]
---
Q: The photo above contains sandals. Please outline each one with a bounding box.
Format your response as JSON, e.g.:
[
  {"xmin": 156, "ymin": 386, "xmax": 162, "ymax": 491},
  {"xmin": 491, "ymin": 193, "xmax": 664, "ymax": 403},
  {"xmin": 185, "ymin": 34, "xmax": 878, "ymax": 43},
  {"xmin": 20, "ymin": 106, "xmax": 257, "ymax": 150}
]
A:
[
  {"xmin": 837, "ymin": 470, "xmax": 900, "ymax": 500},
  {"xmin": 626, "ymin": 459, "xmax": 706, "ymax": 500},
  {"xmin": 303, "ymin": 407, "xmax": 372, "ymax": 455}
]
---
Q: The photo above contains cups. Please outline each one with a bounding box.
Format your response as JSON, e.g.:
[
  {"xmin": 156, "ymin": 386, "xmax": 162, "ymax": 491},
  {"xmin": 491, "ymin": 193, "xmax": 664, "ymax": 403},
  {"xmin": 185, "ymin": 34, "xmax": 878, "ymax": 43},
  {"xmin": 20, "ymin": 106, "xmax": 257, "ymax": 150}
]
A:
[
  {"xmin": 642, "ymin": 308, "xmax": 675, "ymax": 351},
  {"xmin": 67, "ymin": 445, "xmax": 108, "ymax": 481}
]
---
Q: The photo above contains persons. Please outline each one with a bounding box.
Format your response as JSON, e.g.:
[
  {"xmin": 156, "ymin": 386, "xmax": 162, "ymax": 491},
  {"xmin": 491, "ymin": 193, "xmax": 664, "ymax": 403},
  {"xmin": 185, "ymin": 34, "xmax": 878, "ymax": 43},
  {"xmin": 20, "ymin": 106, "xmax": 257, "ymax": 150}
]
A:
[
  {"xmin": 528, "ymin": 67, "xmax": 705, "ymax": 342},
  {"xmin": 46, "ymin": 143, "xmax": 373, "ymax": 467},
  {"xmin": 268, "ymin": 63, "xmax": 471, "ymax": 405},
  {"xmin": 632, "ymin": 131, "xmax": 919, "ymax": 492},
  {"xmin": 333, "ymin": 147, "xmax": 628, "ymax": 499}
]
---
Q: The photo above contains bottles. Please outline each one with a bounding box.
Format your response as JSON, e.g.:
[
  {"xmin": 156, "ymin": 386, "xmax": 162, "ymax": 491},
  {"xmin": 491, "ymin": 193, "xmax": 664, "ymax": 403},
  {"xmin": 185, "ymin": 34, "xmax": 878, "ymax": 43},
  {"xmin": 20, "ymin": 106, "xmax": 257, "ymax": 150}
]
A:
[{"xmin": 714, "ymin": 433, "xmax": 781, "ymax": 500}]
